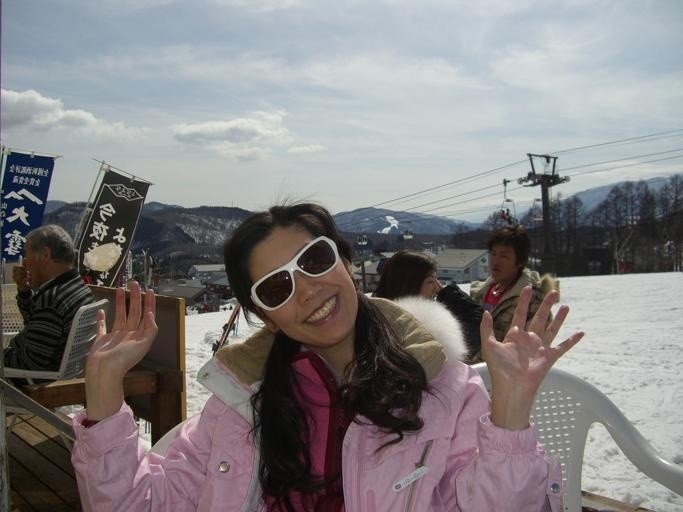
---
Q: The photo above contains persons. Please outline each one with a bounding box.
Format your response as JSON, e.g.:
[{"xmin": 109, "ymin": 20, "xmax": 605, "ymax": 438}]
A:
[
  {"xmin": 2, "ymin": 223, "xmax": 98, "ymax": 395},
  {"xmin": 69, "ymin": 202, "xmax": 585, "ymax": 510},
  {"xmin": 468, "ymin": 224, "xmax": 556, "ymax": 344},
  {"xmin": 506, "ymin": 209, "xmax": 518, "ymax": 223},
  {"xmin": 370, "ymin": 250, "xmax": 486, "ymax": 367},
  {"xmin": 500, "ymin": 210, "xmax": 512, "ymax": 225}
]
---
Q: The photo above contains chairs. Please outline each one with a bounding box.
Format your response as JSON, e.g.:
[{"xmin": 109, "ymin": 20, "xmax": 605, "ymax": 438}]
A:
[
  {"xmin": 0, "ymin": 284, "xmax": 31, "ymax": 349},
  {"xmin": 2, "ymin": 299, "xmax": 110, "ymax": 382},
  {"xmin": 460, "ymin": 362, "xmax": 683, "ymax": 511}
]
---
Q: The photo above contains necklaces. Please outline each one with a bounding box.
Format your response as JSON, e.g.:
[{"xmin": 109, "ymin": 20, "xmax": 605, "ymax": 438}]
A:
[{"xmin": 492, "ymin": 276, "xmax": 517, "ymax": 296}]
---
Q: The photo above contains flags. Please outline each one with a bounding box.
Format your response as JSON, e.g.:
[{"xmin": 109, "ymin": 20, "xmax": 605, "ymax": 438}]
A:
[
  {"xmin": 0, "ymin": 152, "xmax": 57, "ymax": 264},
  {"xmin": 72, "ymin": 169, "xmax": 149, "ymax": 289}
]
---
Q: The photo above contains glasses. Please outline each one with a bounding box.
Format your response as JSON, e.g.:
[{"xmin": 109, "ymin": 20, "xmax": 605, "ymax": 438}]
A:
[{"xmin": 251, "ymin": 236, "xmax": 339, "ymax": 312}]
